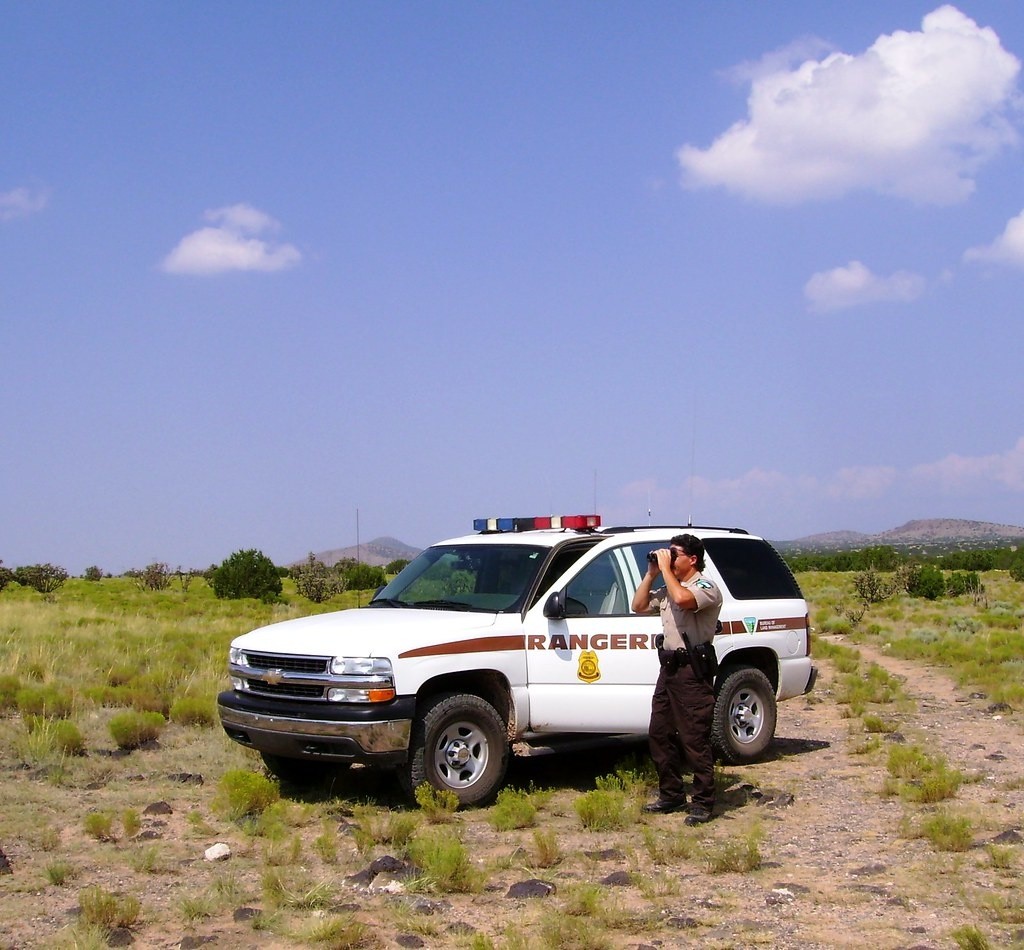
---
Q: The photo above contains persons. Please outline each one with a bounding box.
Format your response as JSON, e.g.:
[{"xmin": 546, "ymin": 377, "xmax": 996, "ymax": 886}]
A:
[{"xmin": 631, "ymin": 535, "xmax": 724, "ymax": 826}]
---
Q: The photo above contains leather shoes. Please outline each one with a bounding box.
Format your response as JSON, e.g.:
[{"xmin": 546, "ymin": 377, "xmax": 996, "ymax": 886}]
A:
[
  {"xmin": 644, "ymin": 798, "xmax": 688, "ymax": 813},
  {"xmin": 684, "ymin": 808, "xmax": 717, "ymax": 826}
]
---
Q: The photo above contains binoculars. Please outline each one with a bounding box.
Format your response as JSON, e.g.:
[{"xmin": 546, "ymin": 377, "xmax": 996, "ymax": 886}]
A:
[{"xmin": 647, "ymin": 547, "xmax": 678, "ymax": 562}]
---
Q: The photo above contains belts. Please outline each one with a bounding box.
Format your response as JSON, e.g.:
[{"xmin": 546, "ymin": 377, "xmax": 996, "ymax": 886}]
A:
[{"xmin": 674, "ymin": 651, "xmax": 697, "ymax": 662}]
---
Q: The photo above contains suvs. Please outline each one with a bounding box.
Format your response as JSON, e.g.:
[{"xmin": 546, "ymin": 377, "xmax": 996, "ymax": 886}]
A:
[{"xmin": 217, "ymin": 515, "xmax": 818, "ymax": 809}]
